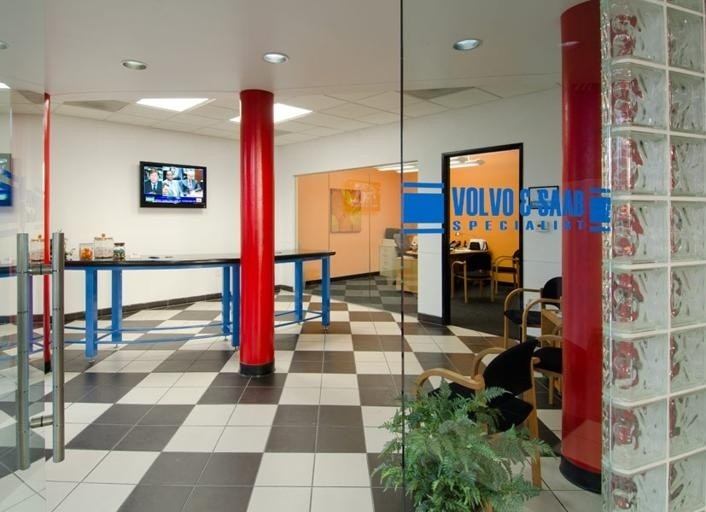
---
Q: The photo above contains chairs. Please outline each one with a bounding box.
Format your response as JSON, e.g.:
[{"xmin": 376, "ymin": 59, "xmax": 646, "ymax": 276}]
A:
[
  {"xmin": 412, "ymin": 276, "xmax": 563, "ymax": 491},
  {"xmin": 490, "ymin": 249, "xmax": 520, "ymax": 302},
  {"xmin": 451, "ymin": 252, "xmax": 495, "ymax": 303}
]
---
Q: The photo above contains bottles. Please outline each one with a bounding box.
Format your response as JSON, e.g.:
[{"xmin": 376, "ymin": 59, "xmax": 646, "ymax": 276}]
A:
[
  {"xmin": 30, "ymin": 235, "xmax": 45, "ymax": 264},
  {"xmin": 80, "ymin": 232, "xmax": 126, "ymax": 261}
]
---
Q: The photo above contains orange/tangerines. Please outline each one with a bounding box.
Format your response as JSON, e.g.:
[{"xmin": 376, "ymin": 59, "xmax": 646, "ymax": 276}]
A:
[{"xmin": 81, "ymin": 248, "xmax": 93, "ymax": 260}]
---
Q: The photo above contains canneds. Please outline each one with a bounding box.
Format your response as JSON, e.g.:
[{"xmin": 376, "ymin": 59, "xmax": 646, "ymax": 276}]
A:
[{"xmin": 112, "ymin": 242, "xmax": 125, "ymax": 260}]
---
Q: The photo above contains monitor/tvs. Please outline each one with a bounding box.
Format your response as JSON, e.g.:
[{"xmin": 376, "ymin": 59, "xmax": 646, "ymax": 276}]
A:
[
  {"xmin": 139, "ymin": 160, "xmax": 207, "ymax": 208},
  {"xmin": 0, "ymin": 152, "xmax": 12, "ymax": 206}
]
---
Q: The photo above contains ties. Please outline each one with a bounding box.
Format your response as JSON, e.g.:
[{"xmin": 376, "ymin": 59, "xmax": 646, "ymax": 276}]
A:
[{"xmin": 153, "ymin": 184, "xmax": 155, "ymax": 190}]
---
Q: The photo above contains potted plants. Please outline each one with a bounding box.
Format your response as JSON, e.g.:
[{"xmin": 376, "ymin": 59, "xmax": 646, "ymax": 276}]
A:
[{"xmin": 367, "ymin": 375, "xmax": 560, "ymax": 512}]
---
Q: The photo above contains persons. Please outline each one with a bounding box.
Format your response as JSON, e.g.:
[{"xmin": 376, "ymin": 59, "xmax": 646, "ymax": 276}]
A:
[{"xmin": 143, "ymin": 168, "xmax": 203, "ymax": 199}]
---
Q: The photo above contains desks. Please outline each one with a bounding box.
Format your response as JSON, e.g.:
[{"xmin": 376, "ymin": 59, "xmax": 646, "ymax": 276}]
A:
[
  {"xmin": 0, "ymin": 250, "xmax": 336, "ymax": 359},
  {"xmin": 395, "ymin": 246, "xmax": 488, "ymax": 293}
]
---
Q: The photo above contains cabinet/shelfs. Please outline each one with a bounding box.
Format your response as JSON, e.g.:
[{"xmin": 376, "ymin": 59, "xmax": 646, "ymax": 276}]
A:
[{"xmin": 378, "ymin": 245, "xmax": 401, "ymax": 278}]
